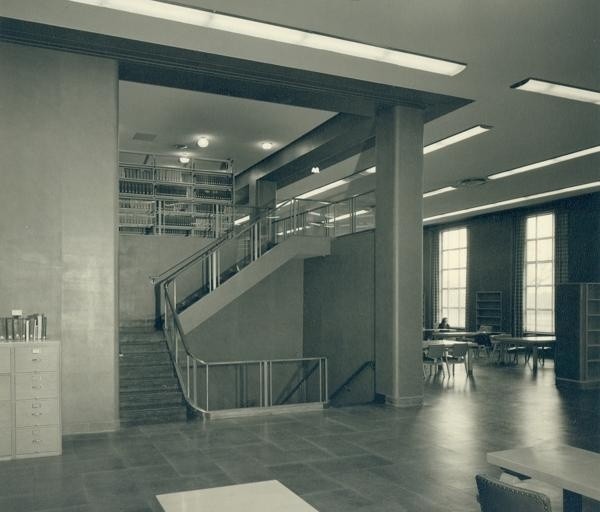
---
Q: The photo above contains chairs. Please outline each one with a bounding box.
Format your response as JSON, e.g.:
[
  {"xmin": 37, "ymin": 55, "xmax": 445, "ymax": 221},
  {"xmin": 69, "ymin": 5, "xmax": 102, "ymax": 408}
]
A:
[
  {"xmin": 471, "ymin": 472, "xmax": 552, "ymax": 512},
  {"xmin": 423, "ymin": 323, "xmax": 557, "ymax": 387}
]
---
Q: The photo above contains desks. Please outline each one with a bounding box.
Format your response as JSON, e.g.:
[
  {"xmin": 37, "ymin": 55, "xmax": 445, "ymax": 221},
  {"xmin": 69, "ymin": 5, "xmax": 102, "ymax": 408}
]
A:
[
  {"xmin": 154, "ymin": 475, "xmax": 320, "ymax": 512},
  {"xmin": 484, "ymin": 440, "xmax": 600, "ymax": 512}
]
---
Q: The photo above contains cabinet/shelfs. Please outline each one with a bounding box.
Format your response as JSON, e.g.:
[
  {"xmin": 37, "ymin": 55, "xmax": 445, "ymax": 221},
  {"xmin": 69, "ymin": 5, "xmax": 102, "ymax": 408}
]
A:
[
  {"xmin": 552, "ymin": 279, "xmax": 600, "ymax": 392},
  {"xmin": 0, "ymin": 341, "xmax": 15, "ymax": 462},
  {"xmin": 13, "ymin": 340, "xmax": 64, "ymax": 458},
  {"xmin": 475, "ymin": 288, "xmax": 505, "ymax": 332},
  {"xmin": 117, "ymin": 159, "xmax": 235, "ymax": 239}
]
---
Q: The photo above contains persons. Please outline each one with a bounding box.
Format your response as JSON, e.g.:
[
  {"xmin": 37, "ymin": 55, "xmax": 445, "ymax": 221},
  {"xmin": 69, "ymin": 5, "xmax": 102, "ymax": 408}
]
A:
[{"xmin": 438, "ymin": 317, "xmax": 450, "ymax": 329}]
